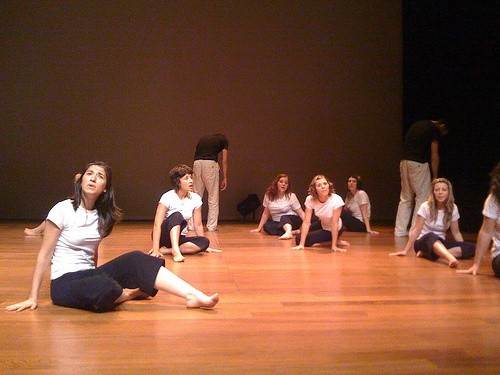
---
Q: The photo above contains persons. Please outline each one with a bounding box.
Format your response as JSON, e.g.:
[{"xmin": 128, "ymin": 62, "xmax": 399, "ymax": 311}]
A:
[
  {"xmin": 340, "ymin": 174, "xmax": 379, "ymax": 235},
  {"xmin": 388, "ymin": 178, "xmax": 474, "ymax": 268},
  {"xmin": 23, "ymin": 172, "xmax": 82, "ymax": 236},
  {"xmin": 249, "ymin": 174, "xmax": 306, "ymax": 240},
  {"xmin": 454, "ymin": 157, "xmax": 500, "ymax": 278},
  {"xmin": 147, "ymin": 164, "xmax": 209, "ymax": 263},
  {"xmin": 289, "ymin": 174, "xmax": 346, "ymax": 253},
  {"xmin": 392, "ymin": 108, "xmax": 452, "ymax": 237},
  {"xmin": 187, "ymin": 130, "xmax": 229, "ymax": 232},
  {"xmin": 3, "ymin": 161, "xmax": 220, "ymax": 313}
]
